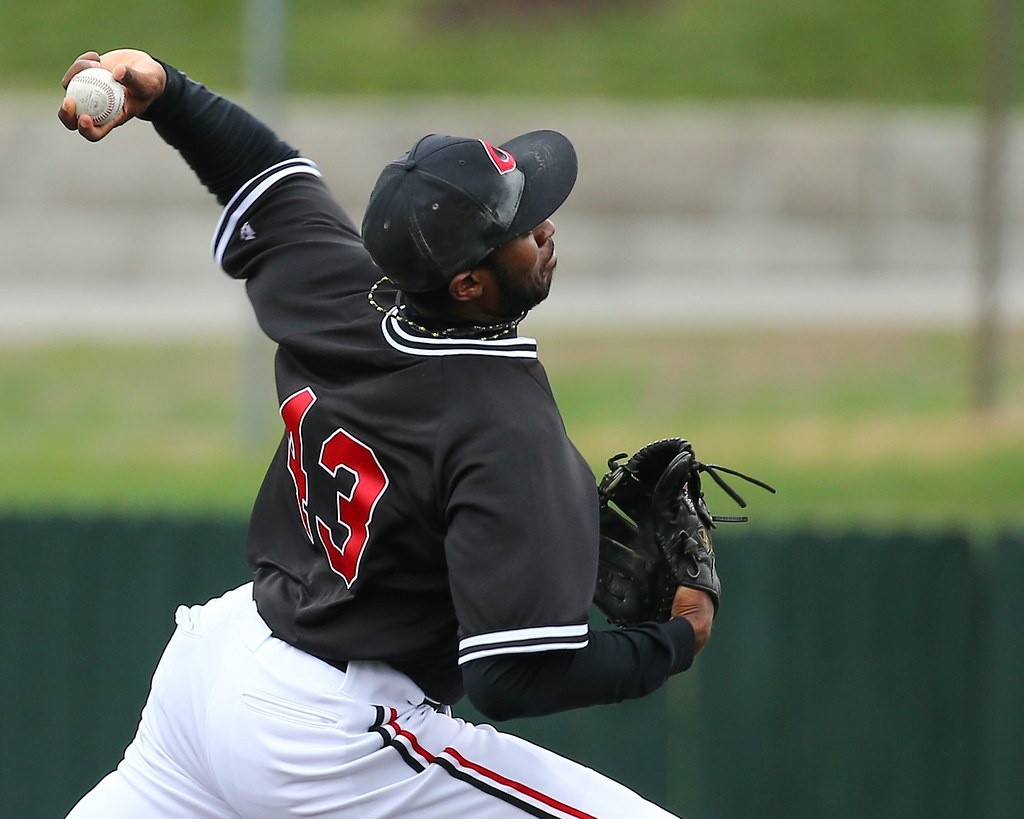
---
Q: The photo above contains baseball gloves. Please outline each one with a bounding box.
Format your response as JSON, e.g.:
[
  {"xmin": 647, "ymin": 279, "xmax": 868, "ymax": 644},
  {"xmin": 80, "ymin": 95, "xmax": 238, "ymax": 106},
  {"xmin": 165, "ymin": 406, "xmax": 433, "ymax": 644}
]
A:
[{"xmin": 594, "ymin": 436, "xmax": 777, "ymax": 634}]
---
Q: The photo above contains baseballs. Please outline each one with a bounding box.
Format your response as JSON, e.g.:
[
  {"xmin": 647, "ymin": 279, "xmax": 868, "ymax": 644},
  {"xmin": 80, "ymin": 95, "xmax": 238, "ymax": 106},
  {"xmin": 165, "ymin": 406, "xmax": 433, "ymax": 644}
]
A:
[{"xmin": 64, "ymin": 66, "xmax": 127, "ymax": 128}]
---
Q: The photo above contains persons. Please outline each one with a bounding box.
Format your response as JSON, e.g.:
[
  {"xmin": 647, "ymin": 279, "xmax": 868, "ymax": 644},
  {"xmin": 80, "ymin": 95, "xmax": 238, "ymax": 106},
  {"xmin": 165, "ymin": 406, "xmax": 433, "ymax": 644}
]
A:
[{"xmin": 57, "ymin": 48, "xmax": 776, "ymax": 819}]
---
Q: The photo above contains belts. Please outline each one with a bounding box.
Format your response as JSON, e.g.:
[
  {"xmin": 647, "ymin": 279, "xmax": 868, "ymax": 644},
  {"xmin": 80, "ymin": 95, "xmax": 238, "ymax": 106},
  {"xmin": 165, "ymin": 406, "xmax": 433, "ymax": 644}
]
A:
[{"xmin": 271, "ymin": 634, "xmax": 445, "ymax": 715}]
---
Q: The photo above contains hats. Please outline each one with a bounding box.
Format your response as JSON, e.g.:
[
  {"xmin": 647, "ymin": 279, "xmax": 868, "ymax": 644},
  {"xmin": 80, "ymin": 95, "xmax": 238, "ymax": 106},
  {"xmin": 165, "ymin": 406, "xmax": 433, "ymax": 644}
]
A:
[{"xmin": 361, "ymin": 130, "xmax": 578, "ymax": 292}]
